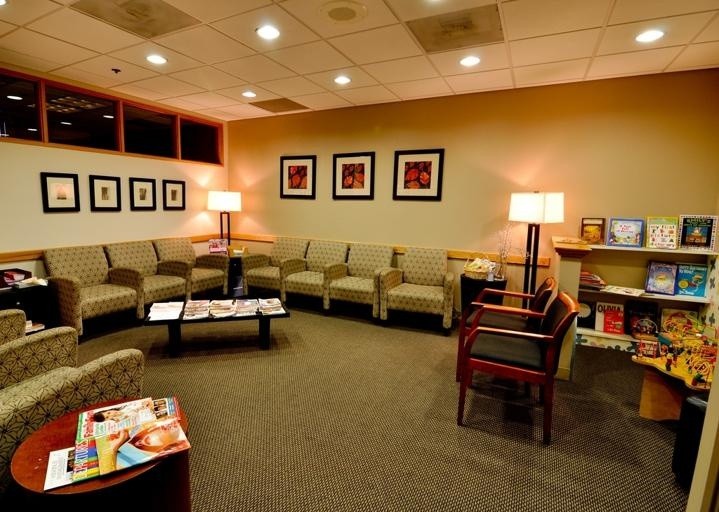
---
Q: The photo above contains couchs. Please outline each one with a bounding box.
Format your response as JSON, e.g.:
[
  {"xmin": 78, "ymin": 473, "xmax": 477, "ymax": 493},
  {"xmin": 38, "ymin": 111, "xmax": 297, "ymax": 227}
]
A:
[
  {"xmin": 325, "ymin": 243, "xmax": 394, "ymax": 317},
  {"xmin": 104, "ymin": 240, "xmax": 190, "ymax": 317},
  {"xmin": 280, "ymin": 240, "xmax": 348, "ymax": 309},
  {"xmin": 380, "ymin": 246, "xmax": 454, "ymax": 328},
  {"xmin": 0, "ymin": 326, "xmax": 142, "ymax": 472},
  {"xmin": 152, "ymin": 237, "xmax": 229, "ymax": 298},
  {"xmin": 38, "ymin": 246, "xmax": 143, "ymax": 336},
  {"xmin": 240, "ymin": 236, "xmax": 310, "ymax": 301}
]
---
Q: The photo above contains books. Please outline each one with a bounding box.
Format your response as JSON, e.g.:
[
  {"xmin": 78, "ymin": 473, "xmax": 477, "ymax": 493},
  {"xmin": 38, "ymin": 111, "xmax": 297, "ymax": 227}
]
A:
[
  {"xmin": 600, "ymin": 284, "xmax": 645, "ymax": 298},
  {"xmin": 4, "ymin": 271, "xmax": 48, "ymax": 288},
  {"xmin": 594, "ymin": 301, "xmax": 623, "ymax": 335},
  {"xmin": 26, "ymin": 323, "xmax": 45, "ymax": 333},
  {"xmin": 234, "ymin": 298, "xmax": 258, "ymax": 317},
  {"xmin": 579, "ymin": 270, "xmax": 606, "ymax": 290},
  {"xmin": 209, "ymin": 300, "xmax": 234, "ymax": 319},
  {"xmin": 624, "ymin": 299, "xmax": 660, "ymax": 336},
  {"xmin": 581, "ymin": 216, "xmax": 606, "ymax": 245},
  {"xmin": 43, "ymin": 439, "xmax": 99, "ymax": 491},
  {"xmin": 661, "ymin": 308, "xmax": 698, "ymax": 332},
  {"xmin": 26, "ymin": 319, "xmax": 33, "ymax": 329},
  {"xmin": 675, "ymin": 262, "xmax": 708, "ymax": 297},
  {"xmin": 678, "ymin": 215, "xmax": 717, "ymax": 250},
  {"xmin": 644, "ymin": 259, "xmax": 677, "ymax": 296},
  {"xmin": 183, "ymin": 300, "xmax": 209, "ymax": 320},
  {"xmin": 148, "ymin": 301, "xmax": 184, "ymax": 321},
  {"xmin": 259, "ymin": 297, "xmax": 285, "ymax": 314}
]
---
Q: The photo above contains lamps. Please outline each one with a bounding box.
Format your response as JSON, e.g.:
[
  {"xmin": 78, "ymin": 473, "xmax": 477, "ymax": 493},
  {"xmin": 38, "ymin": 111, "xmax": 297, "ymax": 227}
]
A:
[
  {"xmin": 507, "ymin": 191, "xmax": 564, "ymax": 314},
  {"xmin": 208, "ymin": 190, "xmax": 241, "ymax": 251}
]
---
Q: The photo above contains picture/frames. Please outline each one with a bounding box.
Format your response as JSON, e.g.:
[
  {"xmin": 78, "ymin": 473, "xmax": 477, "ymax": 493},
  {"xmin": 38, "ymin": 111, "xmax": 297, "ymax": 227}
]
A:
[
  {"xmin": 162, "ymin": 179, "xmax": 185, "ymax": 210},
  {"xmin": 128, "ymin": 177, "xmax": 156, "ymax": 210},
  {"xmin": 280, "ymin": 155, "xmax": 317, "ymax": 200},
  {"xmin": 89, "ymin": 174, "xmax": 121, "ymax": 211},
  {"xmin": 392, "ymin": 148, "xmax": 445, "ymax": 202},
  {"xmin": 333, "ymin": 152, "xmax": 374, "ymax": 200},
  {"xmin": 40, "ymin": 172, "xmax": 81, "ymax": 212}
]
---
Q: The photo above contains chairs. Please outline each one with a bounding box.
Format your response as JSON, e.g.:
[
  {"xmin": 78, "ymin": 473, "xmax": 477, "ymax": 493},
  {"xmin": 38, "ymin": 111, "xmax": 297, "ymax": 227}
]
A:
[
  {"xmin": 458, "ymin": 288, "xmax": 580, "ymax": 446},
  {"xmin": 455, "ymin": 276, "xmax": 557, "ymax": 381}
]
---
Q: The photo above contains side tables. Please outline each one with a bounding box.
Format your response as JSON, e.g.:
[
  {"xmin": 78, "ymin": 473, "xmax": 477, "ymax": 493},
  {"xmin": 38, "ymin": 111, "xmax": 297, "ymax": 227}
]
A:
[{"xmin": 228, "ymin": 253, "xmax": 243, "ymax": 296}]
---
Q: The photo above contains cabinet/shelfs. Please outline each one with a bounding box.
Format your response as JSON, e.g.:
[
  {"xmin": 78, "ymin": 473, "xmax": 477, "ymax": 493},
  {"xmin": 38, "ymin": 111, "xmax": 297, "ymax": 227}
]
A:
[
  {"xmin": 551, "ymin": 237, "xmax": 719, "ymax": 381},
  {"xmin": 461, "ymin": 272, "xmax": 507, "ymax": 321},
  {"xmin": 0, "ymin": 273, "xmax": 60, "ymax": 332}
]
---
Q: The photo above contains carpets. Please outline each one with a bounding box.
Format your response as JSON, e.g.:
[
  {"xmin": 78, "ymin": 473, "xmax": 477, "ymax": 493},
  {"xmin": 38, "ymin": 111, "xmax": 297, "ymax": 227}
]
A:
[{"xmin": 77, "ymin": 305, "xmax": 690, "ymax": 512}]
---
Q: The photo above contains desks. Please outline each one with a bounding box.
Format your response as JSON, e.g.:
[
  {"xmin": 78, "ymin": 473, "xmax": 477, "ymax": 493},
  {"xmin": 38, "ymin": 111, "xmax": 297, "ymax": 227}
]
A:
[
  {"xmin": 631, "ymin": 333, "xmax": 718, "ymax": 421},
  {"xmin": 11, "ymin": 397, "xmax": 192, "ymax": 512}
]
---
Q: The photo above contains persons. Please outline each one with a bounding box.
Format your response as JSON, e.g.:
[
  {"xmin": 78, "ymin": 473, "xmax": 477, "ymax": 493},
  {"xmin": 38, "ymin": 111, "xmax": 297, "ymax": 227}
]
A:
[
  {"xmin": 154, "ymin": 405, "xmax": 166, "ymax": 412},
  {"xmin": 217, "ymin": 241, "xmax": 223, "ymax": 248},
  {"xmin": 92, "ymin": 401, "xmax": 150, "ymax": 429},
  {"xmin": 98, "ymin": 422, "xmax": 187, "ymax": 475},
  {"xmin": 156, "ymin": 411, "xmax": 168, "ymax": 418},
  {"xmin": 209, "ymin": 244, "xmax": 212, "ymax": 250},
  {"xmin": 213, "ymin": 242, "xmax": 217, "ymax": 248},
  {"xmin": 153, "ymin": 400, "xmax": 168, "ymax": 405}
]
